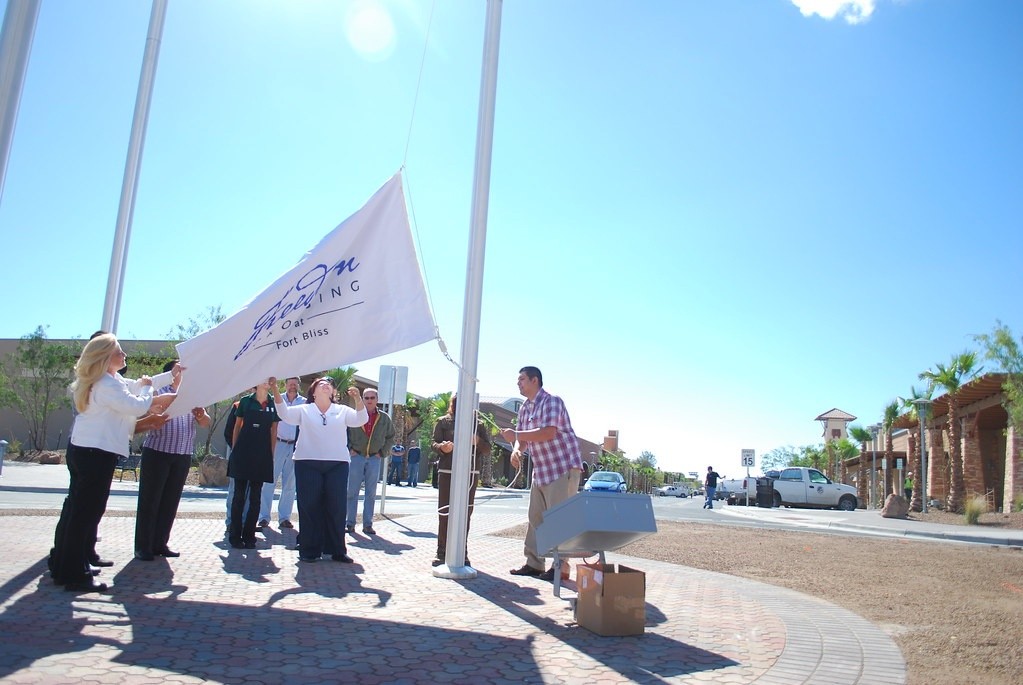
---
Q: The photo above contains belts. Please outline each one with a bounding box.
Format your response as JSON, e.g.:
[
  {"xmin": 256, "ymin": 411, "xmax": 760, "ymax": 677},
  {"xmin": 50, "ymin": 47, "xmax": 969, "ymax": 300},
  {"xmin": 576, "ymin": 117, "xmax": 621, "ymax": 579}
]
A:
[{"xmin": 277, "ymin": 437, "xmax": 295, "ymax": 444}]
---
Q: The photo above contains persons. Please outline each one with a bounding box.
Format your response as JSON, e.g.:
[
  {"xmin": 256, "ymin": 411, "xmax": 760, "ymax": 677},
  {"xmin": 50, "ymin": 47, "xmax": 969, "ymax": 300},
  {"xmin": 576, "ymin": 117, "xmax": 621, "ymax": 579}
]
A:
[
  {"xmin": 432, "ymin": 394, "xmax": 491, "ymax": 568},
  {"xmin": 406, "ymin": 440, "xmax": 420, "ymax": 486},
  {"xmin": 269, "ymin": 378, "xmax": 368, "ymax": 563},
  {"xmin": 294, "ymin": 376, "xmax": 350, "ymax": 548},
  {"xmin": 904, "ymin": 472, "xmax": 914, "ymax": 500},
  {"xmin": 259, "ymin": 376, "xmax": 307, "ymax": 528},
  {"xmin": 49, "ymin": 330, "xmax": 186, "ymax": 590},
  {"xmin": 690, "ymin": 488, "xmax": 693, "ymax": 498},
  {"xmin": 134, "ymin": 361, "xmax": 211, "ymax": 563},
  {"xmin": 386, "ymin": 438, "xmax": 405, "ymax": 486},
  {"xmin": 224, "ymin": 384, "xmax": 281, "ymax": 549},
  {"xmin": 500, "ymin": 366, "xmax": 582, "ymax": 580},
  {"xmin": 703, "ymin": 466, "xmax": 726, "ymax": 509},
  {"xmin": 345, "ymin": 388, "xmax": 396, "ymax": 534}
]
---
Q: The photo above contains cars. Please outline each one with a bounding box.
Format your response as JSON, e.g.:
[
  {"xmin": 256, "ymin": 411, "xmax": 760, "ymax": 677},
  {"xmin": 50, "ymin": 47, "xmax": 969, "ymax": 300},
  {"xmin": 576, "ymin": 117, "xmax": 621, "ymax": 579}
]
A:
[
  {"xmin": 583, "ymin": 471, "xmax": 627, "ymax": 493},
  {"xmin": 658, "ymin": 482, "xmax": 725, "ymax": 502}
]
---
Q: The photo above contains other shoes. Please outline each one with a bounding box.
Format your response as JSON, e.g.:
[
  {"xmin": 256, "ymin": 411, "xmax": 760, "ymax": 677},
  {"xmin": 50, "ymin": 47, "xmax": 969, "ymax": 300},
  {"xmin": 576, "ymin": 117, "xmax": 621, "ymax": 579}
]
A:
[
  {"xmin": 156, "ymin": 549, "xmax": 180, "ymax": 557},
  {"xmin": 433, "ymin": 552, "xmax": 445, "ymax": 566},
  {"xmin": 396, "ymin": 483, "xmax": 402, "ymax": 487},
  {"xmin": 406, "ymin": 483, "xmax": 411, "ymax": 486},
  {"xmin": 259, "ymin": 520, "xmax": 268, "ymax": 527},
  {"xmin": 539, "ymin": 569, "xmax": 569, "ymax": 581},
  {"xmin": 246, "ymin": 541, "xmax": 255, "ymax": 549},
  {"xmin": 332, "ymin": 553, "xmax": 353, "ymax": 563},
  {"xmin": 703, "ymin": 502, "xmax": 706, "ymax": 509},
  {"xmin": 302, "ymin": 556, "xmax": 314, "ymax": 562},
  {"xmin": 345, "ymin": 527, "xmax": 355, "ymax": 532},
  {"xmin": 48, "ymin": 551, "xmax": 114, "ymax": 592},
  {"xmin": 135, "ymin": 551, "xmax": 154, "ymax": 561},
  {"xmin": 464, "ymin": 556, "xmax": 470, "ymax": 566},
  {"xmin": 510, "ymin": 564, "xmax": 544, "ymax": 575},
  {"xmin": 280, "ymin": 520, "xmax": 293, "ymax": 528},
  {"xmin": 364, "ymin": 527, "xmax": 375, "ymax": 534},
  {"xmin": 707, "ymin": 507, "xmax": 713, "ymax": 509},
  {"xmin": 232, "ymin": 541, "xmax": 245, "ymax": 549}
]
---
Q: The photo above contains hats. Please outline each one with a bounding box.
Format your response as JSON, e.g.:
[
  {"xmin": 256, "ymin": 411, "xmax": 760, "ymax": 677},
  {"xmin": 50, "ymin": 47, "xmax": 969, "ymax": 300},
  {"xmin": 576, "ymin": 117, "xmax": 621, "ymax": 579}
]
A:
[{"xmin": 907, "ymin": 472, "xmax": 913, "ymax": 475}]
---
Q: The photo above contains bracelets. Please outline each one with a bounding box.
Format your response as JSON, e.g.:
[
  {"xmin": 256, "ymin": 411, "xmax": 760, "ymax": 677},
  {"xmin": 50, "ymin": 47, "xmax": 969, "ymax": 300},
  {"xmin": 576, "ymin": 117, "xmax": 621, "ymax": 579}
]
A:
[
  {"xmin": 198, "ymin": 415, "xmax": 205, "ymax": 421},
  {"xmin": 516, "ymin": 431, "xmax": 518, "ymax": 440}
]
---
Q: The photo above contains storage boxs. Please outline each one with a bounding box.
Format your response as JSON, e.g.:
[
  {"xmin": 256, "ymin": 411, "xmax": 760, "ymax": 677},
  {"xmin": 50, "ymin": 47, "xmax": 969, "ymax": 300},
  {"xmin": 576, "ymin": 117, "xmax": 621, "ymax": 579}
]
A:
[{"xmin": 576, "ymin": 564, "xmax": 646, "ymax": 636}]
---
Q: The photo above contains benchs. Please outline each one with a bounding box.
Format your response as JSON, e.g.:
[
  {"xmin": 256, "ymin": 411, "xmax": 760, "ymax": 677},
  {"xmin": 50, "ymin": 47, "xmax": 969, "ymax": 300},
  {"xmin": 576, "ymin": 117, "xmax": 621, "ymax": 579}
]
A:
[{"xmin": 116, "ymin": 455, "xmax": 141, "ymax": 483}]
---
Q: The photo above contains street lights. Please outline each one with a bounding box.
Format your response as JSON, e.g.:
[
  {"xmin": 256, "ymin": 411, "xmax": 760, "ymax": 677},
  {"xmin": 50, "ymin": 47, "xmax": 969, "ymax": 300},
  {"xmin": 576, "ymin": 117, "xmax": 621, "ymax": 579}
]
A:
[
  {"xmin": 865, "ymin": 424, "xmax": 882, "ymax": 508},
  {"xmin": 908, "ymin": 397, "xmax": 934, "ymax": 513},
  {"xmin": 589, "ymin": 452, "xmax": 597, "ymax": 476}
]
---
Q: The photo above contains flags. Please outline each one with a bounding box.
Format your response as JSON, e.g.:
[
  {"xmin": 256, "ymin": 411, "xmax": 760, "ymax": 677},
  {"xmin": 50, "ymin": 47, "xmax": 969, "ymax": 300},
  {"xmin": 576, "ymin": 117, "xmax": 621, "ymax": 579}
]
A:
[{"xmin": 164, "ymin": 172, "xmax": 437, "ymax": 420}]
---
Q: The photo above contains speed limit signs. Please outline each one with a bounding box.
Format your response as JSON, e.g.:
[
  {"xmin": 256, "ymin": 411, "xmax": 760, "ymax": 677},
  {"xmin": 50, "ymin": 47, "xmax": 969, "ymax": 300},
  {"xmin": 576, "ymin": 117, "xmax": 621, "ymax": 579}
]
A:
[{"xmin": 741, "ymin": 449, "xmax": 755, "ymax": 466}]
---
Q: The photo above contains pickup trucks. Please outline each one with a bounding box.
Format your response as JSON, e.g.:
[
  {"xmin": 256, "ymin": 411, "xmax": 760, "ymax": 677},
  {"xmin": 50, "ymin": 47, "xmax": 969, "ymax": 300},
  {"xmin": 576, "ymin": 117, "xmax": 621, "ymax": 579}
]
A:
[{"xmin": 721, "ymin": 467, "xmax": 858, "ymax": 512}]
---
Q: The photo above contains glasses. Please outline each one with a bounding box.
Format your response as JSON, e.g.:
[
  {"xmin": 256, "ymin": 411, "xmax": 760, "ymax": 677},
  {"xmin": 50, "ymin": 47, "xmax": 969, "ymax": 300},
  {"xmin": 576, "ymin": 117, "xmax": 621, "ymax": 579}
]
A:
[
  {"xmin": 364, "ymin": 397, "xmax": 375, "ymax": 400},
  {"xmin": 321, "ymin": 413, "xmax": 327, "ymax": 425}
]
